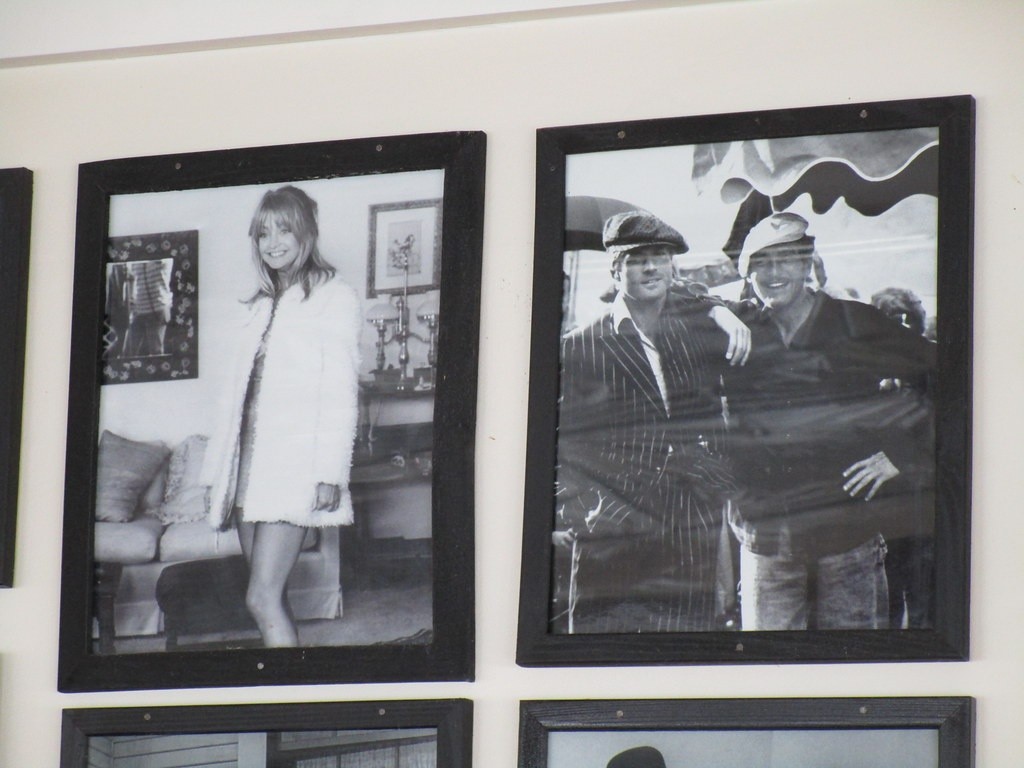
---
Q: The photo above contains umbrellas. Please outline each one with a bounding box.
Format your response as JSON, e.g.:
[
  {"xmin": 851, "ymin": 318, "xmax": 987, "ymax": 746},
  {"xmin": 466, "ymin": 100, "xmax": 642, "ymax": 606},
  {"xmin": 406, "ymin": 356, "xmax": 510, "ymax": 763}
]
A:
[{"xmin": 565, "ymin": 196, "xmax": 655, "ymax": 251}]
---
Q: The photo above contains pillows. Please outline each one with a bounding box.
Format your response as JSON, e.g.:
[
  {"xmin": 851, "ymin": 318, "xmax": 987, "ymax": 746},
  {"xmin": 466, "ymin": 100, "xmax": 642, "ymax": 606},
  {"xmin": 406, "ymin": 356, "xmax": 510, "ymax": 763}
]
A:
[
  {"xmin": 94, "ymin": 429, "xmax": 171, "ymax": 524},
  {"xmin": 181, "ymin": 441, "xmax": 205, "ymax": 491}
]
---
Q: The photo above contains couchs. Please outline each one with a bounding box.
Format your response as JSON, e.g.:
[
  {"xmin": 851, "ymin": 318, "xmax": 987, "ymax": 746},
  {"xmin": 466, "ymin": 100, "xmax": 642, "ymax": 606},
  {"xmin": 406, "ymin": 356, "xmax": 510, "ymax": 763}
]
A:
[{"xmin": 91, "ymin": 434, "xmax": 343, "ymax": 640}]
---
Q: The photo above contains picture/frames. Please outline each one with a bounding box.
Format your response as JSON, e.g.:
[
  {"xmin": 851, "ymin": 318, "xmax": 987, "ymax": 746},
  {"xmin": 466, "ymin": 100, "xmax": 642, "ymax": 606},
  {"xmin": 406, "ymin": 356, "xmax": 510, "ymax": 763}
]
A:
[
  {"xmin": 59, "ymin": 698, "xmax": 473, "ymax": 768},
  {"xmin": 101, "ymin": 230, "xmax": 199, "ymax": 386},
  {"xmin": 514, "ymin": 95, "xmax": 973, "ymax": 670},
  {"xmin": 57, "ymin": 131, "xmax": 486, "ymax": 693},
  {"xmin": 366, "ymin": 197, "xmax": 443, "ymax": 297},
  {"xmin": 517, "ymin": 696, "xmax": 976, "ymax": 768}
]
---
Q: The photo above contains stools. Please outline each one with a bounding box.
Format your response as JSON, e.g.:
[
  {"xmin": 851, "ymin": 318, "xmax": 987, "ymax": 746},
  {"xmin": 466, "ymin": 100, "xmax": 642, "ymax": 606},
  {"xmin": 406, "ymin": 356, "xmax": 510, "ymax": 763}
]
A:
[{"xmin": 155, "ymin": 555, "xmax": 264, "ymax": 651}]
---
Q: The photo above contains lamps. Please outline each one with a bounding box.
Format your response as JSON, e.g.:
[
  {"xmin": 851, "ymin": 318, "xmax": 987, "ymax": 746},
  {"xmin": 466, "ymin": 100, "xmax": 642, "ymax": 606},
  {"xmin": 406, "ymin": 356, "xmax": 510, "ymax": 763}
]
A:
[{"xmin": 364, "ymin": 255, "xmax": 440, "ymax": 394}]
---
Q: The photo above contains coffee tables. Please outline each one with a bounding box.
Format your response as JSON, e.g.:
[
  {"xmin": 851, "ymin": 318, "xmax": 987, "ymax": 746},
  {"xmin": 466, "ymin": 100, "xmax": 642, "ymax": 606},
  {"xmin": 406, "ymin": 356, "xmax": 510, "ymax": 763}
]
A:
[{"xmin": 91, "ymin": 561, "xmax": 123, "ymax": 654}]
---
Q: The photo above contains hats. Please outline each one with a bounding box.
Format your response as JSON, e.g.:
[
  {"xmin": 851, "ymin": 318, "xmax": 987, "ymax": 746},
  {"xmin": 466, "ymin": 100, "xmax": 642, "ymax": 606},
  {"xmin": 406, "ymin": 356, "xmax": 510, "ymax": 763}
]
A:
[
  {"xmin": 738, "ymin": 211, "xmax": 815, "ymax": 277},
  {"xmin": 602, "ymin": 209, "xmax": 690, "ymax": 261}
]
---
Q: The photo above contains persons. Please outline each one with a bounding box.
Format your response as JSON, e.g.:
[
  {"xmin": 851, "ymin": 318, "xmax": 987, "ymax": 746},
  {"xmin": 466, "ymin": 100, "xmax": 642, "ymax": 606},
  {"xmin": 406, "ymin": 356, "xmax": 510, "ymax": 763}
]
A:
[
  {"xmin": 550, "ymin": 211, "xmax": 937, "ymax": 633},
  {"xmin": 199, "ymin": 186, "xmax": 359, "ymax": 648}
]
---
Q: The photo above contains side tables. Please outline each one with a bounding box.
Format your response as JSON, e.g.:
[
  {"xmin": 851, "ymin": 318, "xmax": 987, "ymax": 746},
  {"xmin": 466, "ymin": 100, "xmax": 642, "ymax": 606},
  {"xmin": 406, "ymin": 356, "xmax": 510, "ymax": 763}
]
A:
[{"xmin": 344, "ymin": 421, "xmax": 434, "ymax": 570}]
